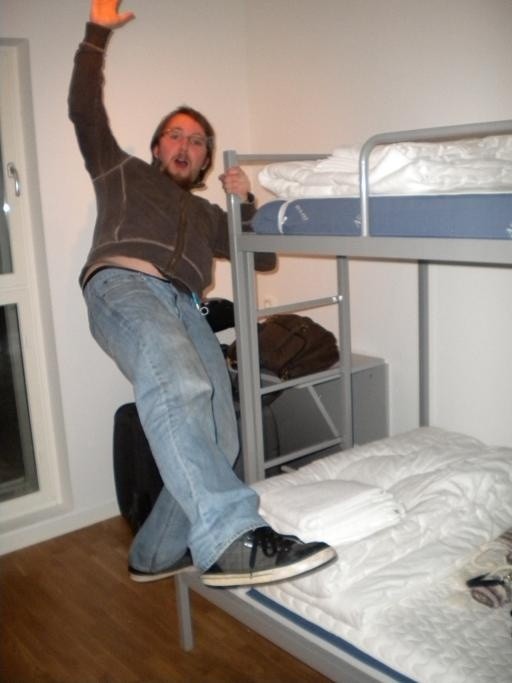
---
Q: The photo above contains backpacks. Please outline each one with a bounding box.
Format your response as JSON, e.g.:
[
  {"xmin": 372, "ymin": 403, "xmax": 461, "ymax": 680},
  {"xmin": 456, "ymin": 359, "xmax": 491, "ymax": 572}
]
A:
[{"xmin": 227, "ymin": 314, "xmax": 339, "ymax": 380}]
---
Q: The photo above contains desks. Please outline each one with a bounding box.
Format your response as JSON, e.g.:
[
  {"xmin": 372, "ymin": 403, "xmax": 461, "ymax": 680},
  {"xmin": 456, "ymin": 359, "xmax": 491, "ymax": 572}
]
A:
[{"xmin": 225, "ymin": 352, "xmax": 389, "ymax": 472}]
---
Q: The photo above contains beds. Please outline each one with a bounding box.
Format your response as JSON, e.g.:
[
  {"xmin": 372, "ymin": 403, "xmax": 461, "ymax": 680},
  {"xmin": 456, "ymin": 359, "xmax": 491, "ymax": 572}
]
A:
[{"xmin": 175, "ymin": 117, "xmax": 510, "ymax": 682}]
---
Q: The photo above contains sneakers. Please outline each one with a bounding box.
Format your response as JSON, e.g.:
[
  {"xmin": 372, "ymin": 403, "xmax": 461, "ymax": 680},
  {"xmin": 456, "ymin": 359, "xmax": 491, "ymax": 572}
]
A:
[{"xmin": 129, "ymin": 527, "xmax": 336, "ymax": 587}]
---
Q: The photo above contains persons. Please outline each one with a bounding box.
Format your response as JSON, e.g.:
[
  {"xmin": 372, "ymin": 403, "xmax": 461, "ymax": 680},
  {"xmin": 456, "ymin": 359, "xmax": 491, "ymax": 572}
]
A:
[{"xmin": 67, "ymin": 0, "xmax": 337, "ymax": 589}]
